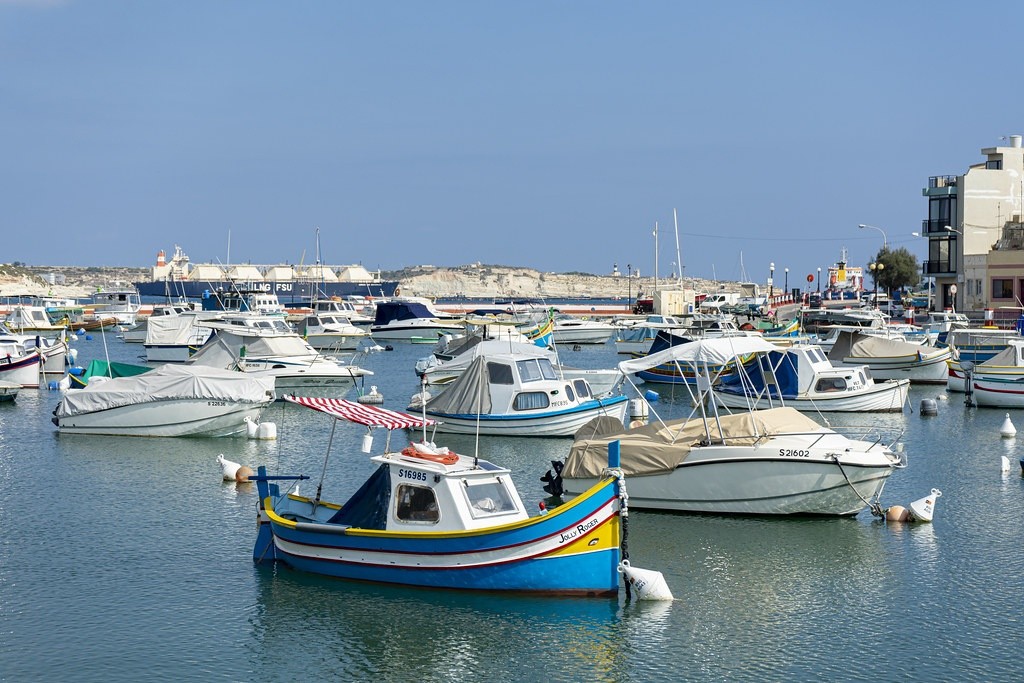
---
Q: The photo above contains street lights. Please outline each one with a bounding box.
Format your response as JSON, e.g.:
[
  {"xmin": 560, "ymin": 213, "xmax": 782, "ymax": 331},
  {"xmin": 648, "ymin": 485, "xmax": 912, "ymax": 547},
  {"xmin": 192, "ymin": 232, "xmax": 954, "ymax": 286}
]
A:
[
  {"xmin": 769, "ymin": 262, "xmax": 776, "ymax": 297},
  {"xmin": 859, "ymin": 223, "xmax": 891, "ymax": 323},
  {"xmin": 784, "ymin": 268, "xmax": 789, "ymax": 294},
  {"xmin": 816, "ymin": 267, "xmax": 822, "ymax": 293},
  {"xmin": 870, "ymin": 263, "xmax": 885, "ymax": 309}
]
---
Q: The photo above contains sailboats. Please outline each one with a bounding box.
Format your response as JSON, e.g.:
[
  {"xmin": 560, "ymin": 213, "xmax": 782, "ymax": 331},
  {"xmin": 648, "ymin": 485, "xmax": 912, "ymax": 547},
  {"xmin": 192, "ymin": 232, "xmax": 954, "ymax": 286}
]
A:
[{"xmin": 0, "ymin": 224, "xmax": 1024, "ymax": 441}]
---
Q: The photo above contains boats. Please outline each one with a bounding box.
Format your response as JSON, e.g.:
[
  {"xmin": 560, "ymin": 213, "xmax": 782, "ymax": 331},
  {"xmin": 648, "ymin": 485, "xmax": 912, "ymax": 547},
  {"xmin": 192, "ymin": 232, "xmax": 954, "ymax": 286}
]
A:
[
  {"xmin": 246, "ymin": 378, "xmax": 631, "ymax": 599},
  {"xmin": 539, "ymin": 323, "xmax": 908, "ymax": 519},
  {"xmin": 47, "ymin": 316, "xmax": 277, "ymax": 441}
]
